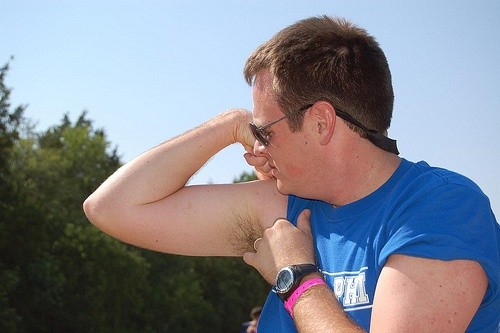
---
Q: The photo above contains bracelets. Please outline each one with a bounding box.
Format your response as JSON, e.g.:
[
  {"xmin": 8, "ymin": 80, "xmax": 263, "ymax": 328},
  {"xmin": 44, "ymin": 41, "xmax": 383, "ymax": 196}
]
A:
[{"xmin": 283, "ymin": 277, "xmax": 329, "ymax": 317}]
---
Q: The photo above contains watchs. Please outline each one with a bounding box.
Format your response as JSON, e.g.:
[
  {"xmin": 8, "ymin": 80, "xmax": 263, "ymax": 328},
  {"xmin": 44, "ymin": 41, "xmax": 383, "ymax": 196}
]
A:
[{"xmin": 275, "ymin": 263, "xmax": 318, "ymax": 301}]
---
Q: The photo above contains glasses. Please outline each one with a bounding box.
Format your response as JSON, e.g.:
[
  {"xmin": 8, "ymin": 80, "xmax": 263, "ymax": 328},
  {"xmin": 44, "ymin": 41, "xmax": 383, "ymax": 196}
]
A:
[{"xmin": 248, "ymin": 103, "xmax": 368, "ymax": 148}]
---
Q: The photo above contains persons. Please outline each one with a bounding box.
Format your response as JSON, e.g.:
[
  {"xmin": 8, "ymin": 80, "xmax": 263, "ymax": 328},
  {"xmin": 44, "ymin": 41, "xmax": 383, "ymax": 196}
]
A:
[
  {"xmin": 246, "ymin": 306, "xmax": 262, "ymax": 333},
  {"xmin": 82, "ymin": 14, "xmax": 500, "ymax": 333}
]
